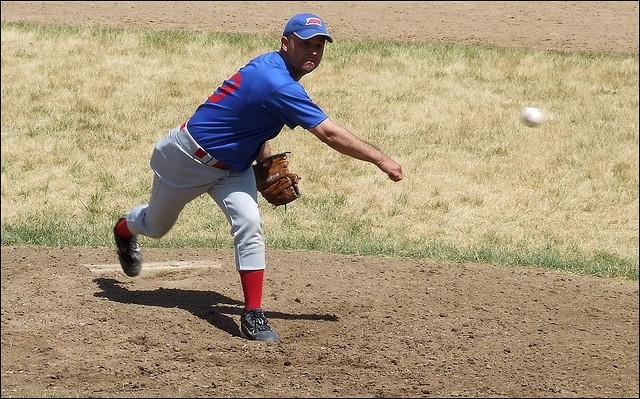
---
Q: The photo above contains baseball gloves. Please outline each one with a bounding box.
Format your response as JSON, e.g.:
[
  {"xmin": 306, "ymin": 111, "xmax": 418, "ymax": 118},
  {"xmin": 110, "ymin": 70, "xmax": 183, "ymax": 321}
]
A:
[{"xmin": 252, "ymin": 151, "xmax": 302, "ymax": 207}]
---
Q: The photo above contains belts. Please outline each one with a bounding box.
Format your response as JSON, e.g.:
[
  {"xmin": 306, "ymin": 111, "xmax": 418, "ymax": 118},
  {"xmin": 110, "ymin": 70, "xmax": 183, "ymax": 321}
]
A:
[{"xmin": 179, "ymin": 121, "xmax": 232, "ymax": 173}]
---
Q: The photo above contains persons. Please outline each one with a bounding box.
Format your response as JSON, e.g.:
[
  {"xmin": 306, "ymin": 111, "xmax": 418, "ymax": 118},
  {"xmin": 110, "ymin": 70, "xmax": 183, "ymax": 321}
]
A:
[{"xmin": 113, "ymin": 14, "xmax": 404, "ymax": 341}]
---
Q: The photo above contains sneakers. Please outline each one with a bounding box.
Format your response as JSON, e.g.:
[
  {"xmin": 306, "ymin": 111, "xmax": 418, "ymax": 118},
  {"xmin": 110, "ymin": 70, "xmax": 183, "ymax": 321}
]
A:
[
  {"xmin": 240, "ymin": 308, "xmax": 280, "ymax": 343},
  {"xmin": 113, "ymin": 214, "xmax": 142, "ymax": 278}
]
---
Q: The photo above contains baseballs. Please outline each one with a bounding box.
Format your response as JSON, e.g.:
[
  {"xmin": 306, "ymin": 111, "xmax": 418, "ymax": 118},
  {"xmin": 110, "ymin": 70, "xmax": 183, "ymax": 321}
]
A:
[{"xmin": 519, "ymin": 107, "xmax": 545, "ymax": 127}]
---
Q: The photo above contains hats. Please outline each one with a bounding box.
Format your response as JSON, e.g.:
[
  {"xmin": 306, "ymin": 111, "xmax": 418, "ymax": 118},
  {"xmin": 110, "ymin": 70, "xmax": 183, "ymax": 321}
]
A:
[{"xmin": 284, "ymin": 13, "xmax": 333, "ymax": 43}]
